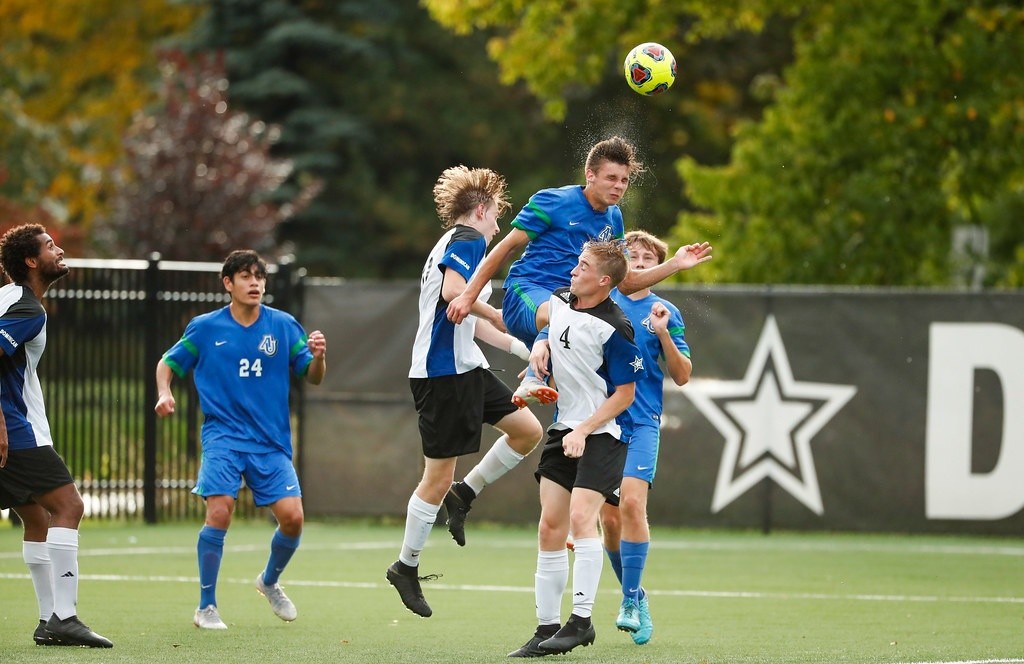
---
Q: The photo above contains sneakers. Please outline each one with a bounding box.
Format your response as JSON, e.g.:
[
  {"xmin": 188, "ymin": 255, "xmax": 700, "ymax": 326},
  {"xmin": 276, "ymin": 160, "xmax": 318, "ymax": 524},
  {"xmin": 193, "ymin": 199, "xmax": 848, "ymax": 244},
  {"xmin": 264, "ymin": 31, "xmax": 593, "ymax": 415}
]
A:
[
  {"xmin": 444, "ymin": 481, "xmax": 472, "ymax": 547},
  {"xmin": 511, "ymin": 376, "xmax": 559, "ymax": 410},
  {"xmin": 616, "ymin": 598, "xmax": 641, "ymax": 633},
  {"xmin": 256, "ymin": 574, "xmax": 297, "ymax": 621},
  {"xmin": 44, "ymin": 612, "xmax": 113, "ymax": 648},
  {"xmin": 33, "ymin": 619, "xmax": 72, "ymax": 646},
  {"xmin": 538, "ymin": 614, "xmax": 596, "ymax": 655},
  {"xmin": 194, "ymin": 604, "xmax": 228, "ymax": 630},
  {"xmin": 506, "ymin": 624, "xmax": 561, "ymax": 657},
  {"xmin": 630, "ymin": 587, "xmax": 653, "ymax": 645},
  {"xmin": 385, "ymin": 560, "xmax": 443, "ymax": 618}
]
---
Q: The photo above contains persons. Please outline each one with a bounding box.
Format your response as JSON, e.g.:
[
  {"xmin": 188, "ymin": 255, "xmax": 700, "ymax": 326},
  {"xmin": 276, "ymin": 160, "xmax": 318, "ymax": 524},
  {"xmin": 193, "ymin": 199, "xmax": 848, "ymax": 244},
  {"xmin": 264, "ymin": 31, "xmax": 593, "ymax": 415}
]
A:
[
  {"xmin": 386, "ymin": 135, "xmax": 713, "ymax": 658},
  {"xmin": 0, "ymin": 223, "xmax": 114, "ymax": 650},
  {"xmin": 153, "ymin": 249, "xmax": 326, "ymax": 629}
]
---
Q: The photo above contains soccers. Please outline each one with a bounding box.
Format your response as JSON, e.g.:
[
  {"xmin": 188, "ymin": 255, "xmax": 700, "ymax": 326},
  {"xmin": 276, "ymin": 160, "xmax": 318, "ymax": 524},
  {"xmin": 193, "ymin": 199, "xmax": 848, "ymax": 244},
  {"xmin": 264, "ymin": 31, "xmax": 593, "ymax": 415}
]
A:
[{"xmin": 624, "ymin": 41, "xmax": 678, "ymax": 97}]
